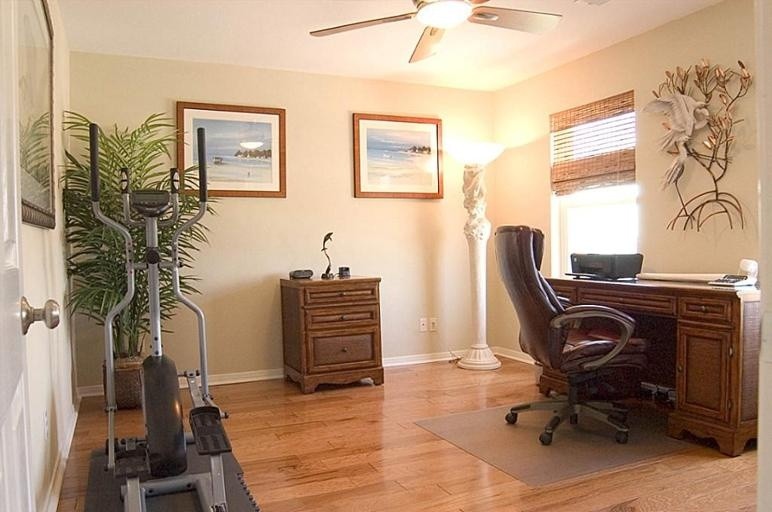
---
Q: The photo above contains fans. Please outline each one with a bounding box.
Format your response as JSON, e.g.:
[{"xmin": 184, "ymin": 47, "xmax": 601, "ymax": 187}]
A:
[{"xmin": 307, "ymin": 0, "xmax": 565, "ymax": 65}]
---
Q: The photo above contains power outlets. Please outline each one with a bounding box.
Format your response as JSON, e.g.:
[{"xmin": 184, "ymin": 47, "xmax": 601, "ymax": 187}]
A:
[{"xmin": 429, "ymin": 318, "xmax": 437, "ymax": 332}]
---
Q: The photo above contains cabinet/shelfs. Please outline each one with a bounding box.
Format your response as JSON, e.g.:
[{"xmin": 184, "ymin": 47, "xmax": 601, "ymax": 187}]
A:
[{"xmin": 280, "ymin": 275, "xmax": 384, "ymax": 393}]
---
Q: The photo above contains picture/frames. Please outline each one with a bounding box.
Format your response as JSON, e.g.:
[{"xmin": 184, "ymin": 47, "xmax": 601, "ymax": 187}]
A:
[
  {"xmin": 8, "ymin": 0, "xmax": 56, "ymax": 231},
  {"xmin": 176, "ymin": 99, "xmax": 286, "ymax": 199},
  {"xmin": 351, "ymin": 114, "xmax": 445, "ymax": 198}
]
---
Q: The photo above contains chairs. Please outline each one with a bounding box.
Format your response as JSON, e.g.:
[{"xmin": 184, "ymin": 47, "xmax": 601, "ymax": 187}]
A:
[{"xmin": 493, "ymin": 226, "xmax": 651, "ymax": 445}]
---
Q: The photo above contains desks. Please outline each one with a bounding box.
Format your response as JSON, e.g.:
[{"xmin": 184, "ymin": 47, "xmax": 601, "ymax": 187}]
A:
[{"xmin": 542, "ymin": 273, "xmax": 761, "ymax": 457}]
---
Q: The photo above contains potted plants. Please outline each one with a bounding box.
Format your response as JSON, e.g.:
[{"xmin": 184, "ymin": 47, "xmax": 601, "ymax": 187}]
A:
[{"xmin": 58, "ymin": 109, "xmax": 221, "ymax": 410}]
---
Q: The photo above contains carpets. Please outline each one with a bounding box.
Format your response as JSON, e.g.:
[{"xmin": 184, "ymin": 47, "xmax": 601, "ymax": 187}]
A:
[
  {"xmin": 85, "ymin": 447, "xmax": 261, "ymax": 512},
  {"xmin": 413, "ymin": 399, "xmax": 697, "ymax": 489}
]
